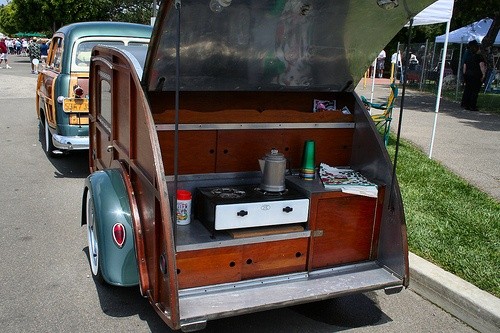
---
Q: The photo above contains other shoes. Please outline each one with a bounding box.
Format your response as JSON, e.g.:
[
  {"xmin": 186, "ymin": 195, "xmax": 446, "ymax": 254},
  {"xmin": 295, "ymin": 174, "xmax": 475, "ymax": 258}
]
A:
[
  {"xmin": 6, "ymin": 65, "xmax": 12, "ymax": 69},
  {"xmin": 461, "ymin": 102, "xmax": 467, "ymax": 106},
  {"xmin": 468, "ymin": 106, "xmax": 478, "ymax": 111}
]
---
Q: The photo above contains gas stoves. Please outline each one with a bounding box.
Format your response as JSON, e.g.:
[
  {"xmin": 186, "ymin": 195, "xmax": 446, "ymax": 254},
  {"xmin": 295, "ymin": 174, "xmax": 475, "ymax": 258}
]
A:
[{"xmin": 192, "ymin": 183, "xmax": 310, "ymax": 235}]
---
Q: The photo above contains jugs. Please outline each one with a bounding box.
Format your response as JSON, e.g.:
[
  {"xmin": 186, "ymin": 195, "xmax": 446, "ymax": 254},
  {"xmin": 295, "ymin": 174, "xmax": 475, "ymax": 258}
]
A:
[{"xmin": 258, "ymin": 149, "xmax": 286, "ymax": 192}]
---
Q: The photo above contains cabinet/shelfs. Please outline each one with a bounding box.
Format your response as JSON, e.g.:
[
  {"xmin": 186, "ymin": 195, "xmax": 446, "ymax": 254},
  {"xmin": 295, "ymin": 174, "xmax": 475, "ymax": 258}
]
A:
[{"xmin": 176, "ymin": 236, "xmax": 308, "ymax": 292}]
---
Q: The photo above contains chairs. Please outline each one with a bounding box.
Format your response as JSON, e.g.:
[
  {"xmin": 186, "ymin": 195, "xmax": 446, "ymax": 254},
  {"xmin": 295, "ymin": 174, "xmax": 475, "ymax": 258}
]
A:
[{"xmin": 361, "ymin": 83, "xmax": 400, "ymax": 146}]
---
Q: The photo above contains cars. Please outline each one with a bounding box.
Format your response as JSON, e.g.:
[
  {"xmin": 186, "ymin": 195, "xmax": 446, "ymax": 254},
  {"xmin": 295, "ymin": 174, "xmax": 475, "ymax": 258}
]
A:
[{"xmin": 31, "ymin": 22, "xmax": 153, "ymax": 161}]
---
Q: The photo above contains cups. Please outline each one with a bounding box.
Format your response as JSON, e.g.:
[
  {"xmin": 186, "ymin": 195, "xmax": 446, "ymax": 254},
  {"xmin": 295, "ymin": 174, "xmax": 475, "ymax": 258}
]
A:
[{"xmin": 301, "ymin": 141, "xmax": 316, "ymax": 182}]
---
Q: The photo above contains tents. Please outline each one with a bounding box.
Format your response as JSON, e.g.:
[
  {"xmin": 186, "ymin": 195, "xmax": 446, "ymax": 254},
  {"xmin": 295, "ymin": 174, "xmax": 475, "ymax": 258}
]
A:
[
  {"xmin": 404, "ymin": 0, "xmax": 455, "ymax": 159},
  {"xmin": 13, "ymin": 32, "xmax": 46, "ymax": 38},
  {"xmin": 427, "ymin": 17, "xmax": 500, "ymax": 88}
]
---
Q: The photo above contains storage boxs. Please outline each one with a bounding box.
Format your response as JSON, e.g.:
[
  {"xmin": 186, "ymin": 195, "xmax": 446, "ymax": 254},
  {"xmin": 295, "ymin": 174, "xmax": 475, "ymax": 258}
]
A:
[{"xmin": 288, "ymin": 169, "xmax": 386, "ymax": 270}]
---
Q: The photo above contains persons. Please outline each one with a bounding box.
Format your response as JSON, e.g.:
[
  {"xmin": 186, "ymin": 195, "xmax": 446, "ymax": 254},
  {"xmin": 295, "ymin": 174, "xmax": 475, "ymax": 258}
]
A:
[
  {"xmin": 365, "ymin": 44, "xmax": 435, "ymax": 80},
  {"xmin": 462, "ymin": 39, "xmax": 487, "ymax": 112},
  {"xmin": 1, "ymin": 36, "xmax": 51, "ymax": 56},
  {"xmin": 28, "ymin": 37, "xmax": 49, "ymax": 74},
  {"xmin": 1, "ymin": 36, "xmax": 12, "ymax": 70}
]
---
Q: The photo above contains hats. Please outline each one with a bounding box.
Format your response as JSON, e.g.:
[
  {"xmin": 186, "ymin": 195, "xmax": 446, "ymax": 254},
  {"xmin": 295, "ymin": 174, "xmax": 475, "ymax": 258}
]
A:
[
  {"xmin": 33, "ymin": 37, "xmax": 37, "ymax": 41},
  {"xmin": 469, "ymin": 40, "xmax": 480, "ymax": 47},
  {"xmin": 42, "ymin": 40, "xmax": 46, "ymax": 44}
]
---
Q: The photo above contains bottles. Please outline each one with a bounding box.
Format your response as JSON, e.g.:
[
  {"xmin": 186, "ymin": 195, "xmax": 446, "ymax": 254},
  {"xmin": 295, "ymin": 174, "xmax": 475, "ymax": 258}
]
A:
[{"xmin": 176, "ymin": 189, "xmax": 192, "ymax": 225}]
